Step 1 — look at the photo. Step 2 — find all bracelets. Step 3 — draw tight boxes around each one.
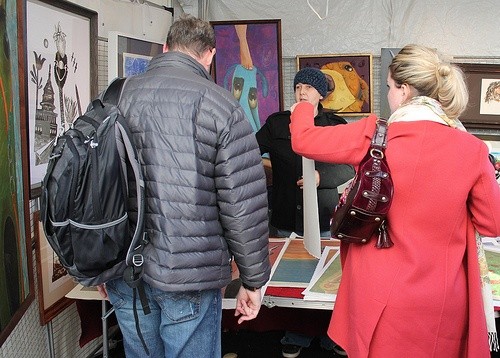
[240,280,262,292]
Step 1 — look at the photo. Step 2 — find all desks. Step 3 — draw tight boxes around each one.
[102,300,335,358]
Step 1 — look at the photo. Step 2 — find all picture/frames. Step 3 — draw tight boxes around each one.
[117,34,164,78]
[24,0,99,200]
[472,134,500,184]
[296,52,374,117]
[209,18,284,160]
[33,210,75,326]
[0,0,36,350]
[449,62,500,130]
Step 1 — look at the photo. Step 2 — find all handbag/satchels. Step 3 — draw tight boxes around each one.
[330,118,395,249]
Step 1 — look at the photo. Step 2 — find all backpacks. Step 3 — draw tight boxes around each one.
[40,76,144,286]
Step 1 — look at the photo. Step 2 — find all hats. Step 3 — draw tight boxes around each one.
[293,68,327,97]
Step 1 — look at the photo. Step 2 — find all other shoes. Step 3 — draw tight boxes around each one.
[282,345,302,358]
[333,345,347,355]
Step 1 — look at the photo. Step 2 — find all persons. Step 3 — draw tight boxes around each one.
[255,66,355,358]
[289,43,500,358]
[85,17,271,358]
